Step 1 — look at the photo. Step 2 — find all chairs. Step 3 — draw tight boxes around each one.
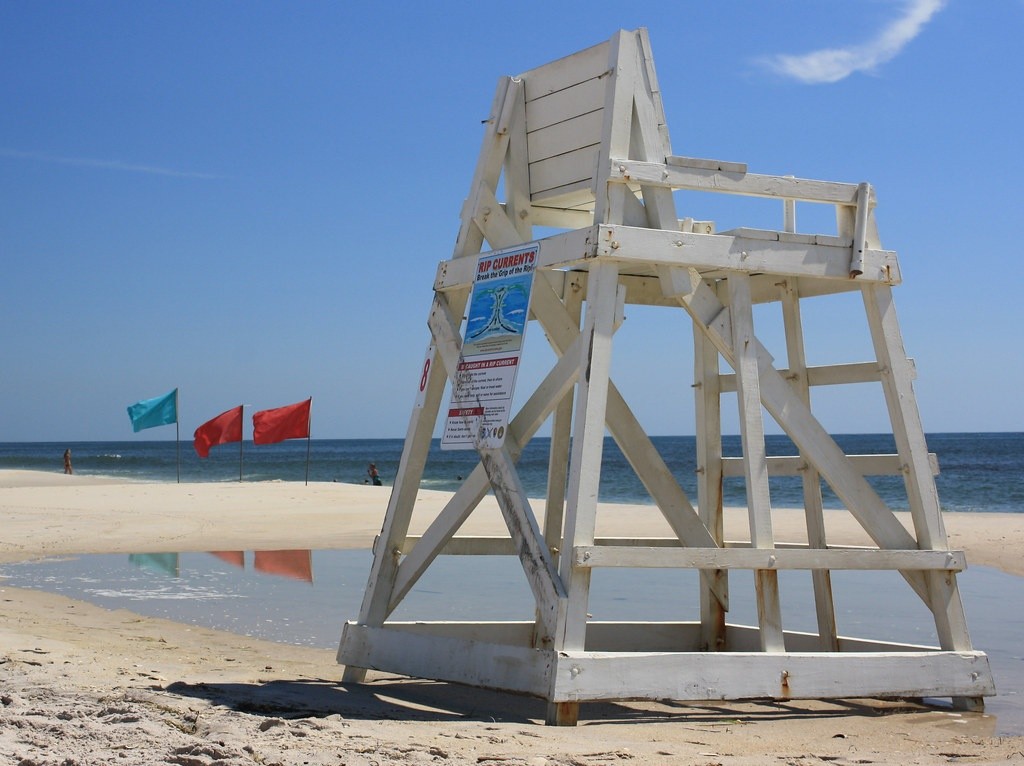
[447,26,880,307]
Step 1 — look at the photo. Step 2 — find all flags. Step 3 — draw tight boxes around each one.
[127,390,177,433]
[130,552,177,577]
[193,405,243,458]
[252,399,310,445]
[254,550,311,580]
[214,550,243,565]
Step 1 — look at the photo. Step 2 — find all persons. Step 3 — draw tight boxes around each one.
[64,448,72,474]
[367,463,382,486]
